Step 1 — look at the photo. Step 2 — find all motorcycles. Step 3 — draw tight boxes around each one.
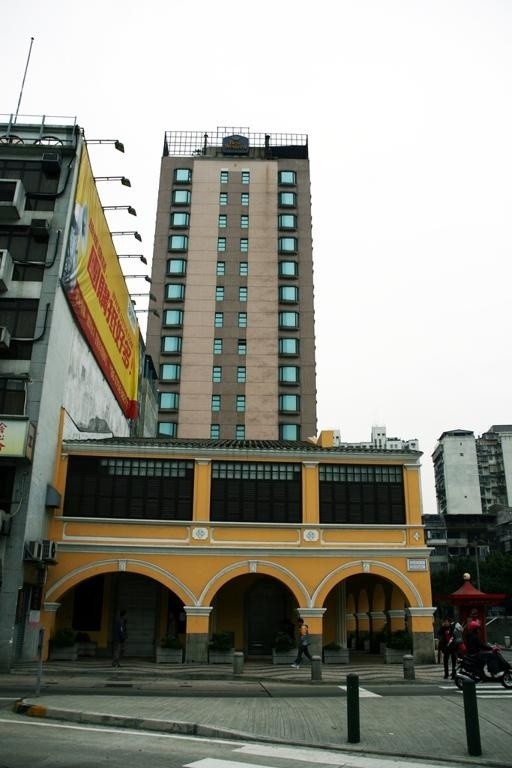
[450,639,512,692]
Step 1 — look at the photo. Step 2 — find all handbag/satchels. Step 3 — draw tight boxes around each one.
[301,627,312,646]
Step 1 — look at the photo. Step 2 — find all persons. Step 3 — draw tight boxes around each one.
[291,617,313,667]
[437,614,459,679]
[463,621,505,678]
[111,607,128,667]
[451,615,465,642]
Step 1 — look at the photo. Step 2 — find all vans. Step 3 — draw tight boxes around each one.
[484,615,512,645]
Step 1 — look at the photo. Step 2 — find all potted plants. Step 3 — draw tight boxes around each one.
[384,634,413,664]
[155,631,236,664]
[272,632,349,665]
[48,628,97,661]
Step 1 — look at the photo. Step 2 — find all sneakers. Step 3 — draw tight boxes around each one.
[289,664,299,669]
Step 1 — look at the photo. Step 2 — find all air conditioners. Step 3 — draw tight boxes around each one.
[29,539,58,562]
